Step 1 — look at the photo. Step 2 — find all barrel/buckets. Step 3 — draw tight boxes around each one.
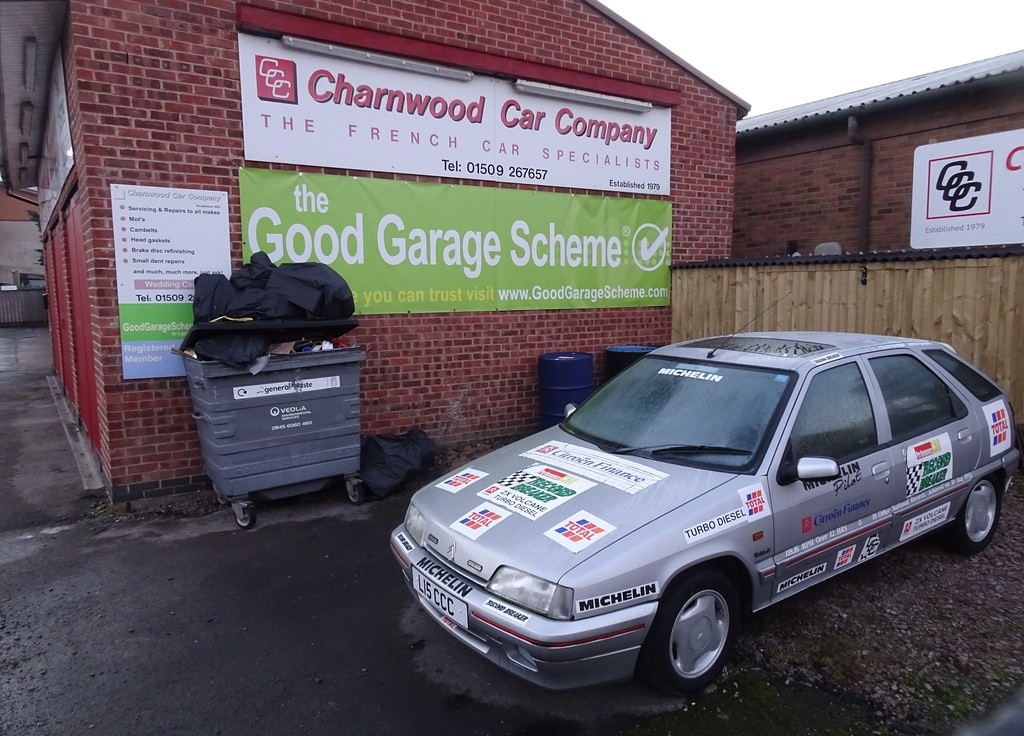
[539,352,595,431]
[604,346,658,383]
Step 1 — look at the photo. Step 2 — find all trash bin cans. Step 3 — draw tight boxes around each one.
[182,342,367,530]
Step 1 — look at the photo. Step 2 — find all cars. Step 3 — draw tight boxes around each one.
[391,326,1020,697]
